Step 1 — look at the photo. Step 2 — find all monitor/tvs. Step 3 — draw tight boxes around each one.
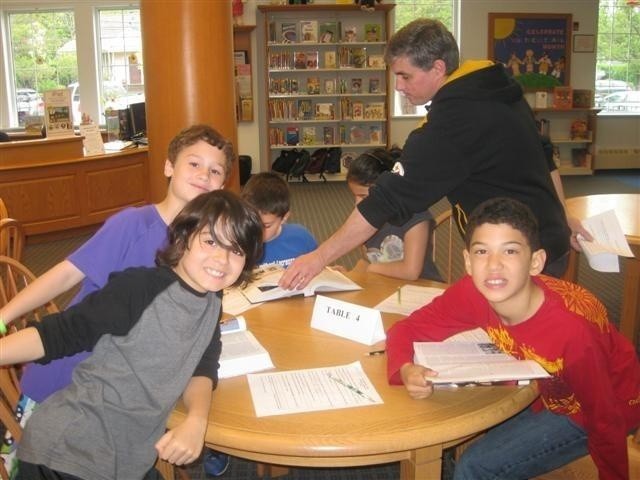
[129,102,146,134]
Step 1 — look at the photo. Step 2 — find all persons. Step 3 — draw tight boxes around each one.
[330,146,444,283]
[0,190,264,480]
[385,196,640,480]
[201,171,320,477]
[276,18,594,290]
[0,124,234,480]
[493,49,565,79]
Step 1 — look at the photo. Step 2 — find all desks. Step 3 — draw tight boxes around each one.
[155,268,539,480]
[561,192,640,341]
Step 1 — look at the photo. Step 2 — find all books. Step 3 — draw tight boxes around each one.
[410,339,554,388]
[217,316,277,380]
[237,264,364,305]
[266,19,386,145]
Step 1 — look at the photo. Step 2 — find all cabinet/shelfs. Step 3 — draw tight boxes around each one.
[528,108,595,175]
[257,4,395,183]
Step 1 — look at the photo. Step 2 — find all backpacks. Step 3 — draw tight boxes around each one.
[272,147,341,184]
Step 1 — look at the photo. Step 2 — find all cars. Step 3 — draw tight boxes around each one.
[15,89,45,115]
[594,80,640,114]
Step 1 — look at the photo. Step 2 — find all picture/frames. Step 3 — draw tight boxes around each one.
[573,34,595,53]
[487,12,573,89]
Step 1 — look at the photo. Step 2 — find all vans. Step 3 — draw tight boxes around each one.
[68,81,126,114]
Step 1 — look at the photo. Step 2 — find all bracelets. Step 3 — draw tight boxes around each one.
[0,319,7,335]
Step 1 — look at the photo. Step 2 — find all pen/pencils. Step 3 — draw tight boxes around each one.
[329,374,375,403]
[398,286,400,304]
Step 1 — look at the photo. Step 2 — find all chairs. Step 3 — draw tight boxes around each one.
[431,208,467,285]
[0,254,138,480]
[0,361,186,480]
[453,432,640,480]
[0,197,28,302]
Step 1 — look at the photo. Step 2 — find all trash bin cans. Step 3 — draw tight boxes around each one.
[238,155,252,185]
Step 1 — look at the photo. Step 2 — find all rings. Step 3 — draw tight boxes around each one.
[298,276,305,282]
